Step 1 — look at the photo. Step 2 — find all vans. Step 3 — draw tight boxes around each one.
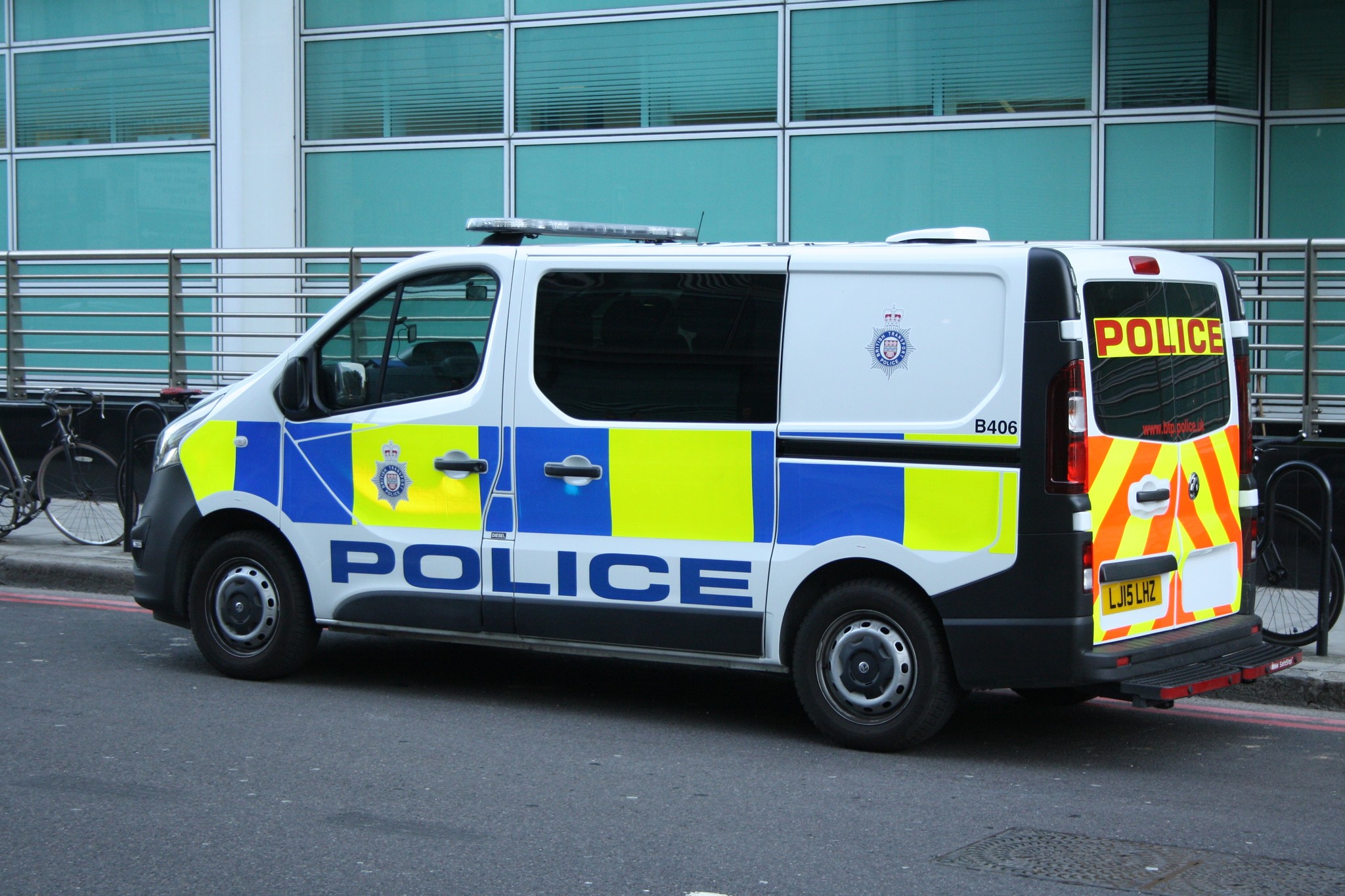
[121,215,1300,754]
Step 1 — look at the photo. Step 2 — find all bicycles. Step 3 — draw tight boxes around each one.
[114,388,202,528]
[0,387,139,546]
[1252,442,1345,649]
